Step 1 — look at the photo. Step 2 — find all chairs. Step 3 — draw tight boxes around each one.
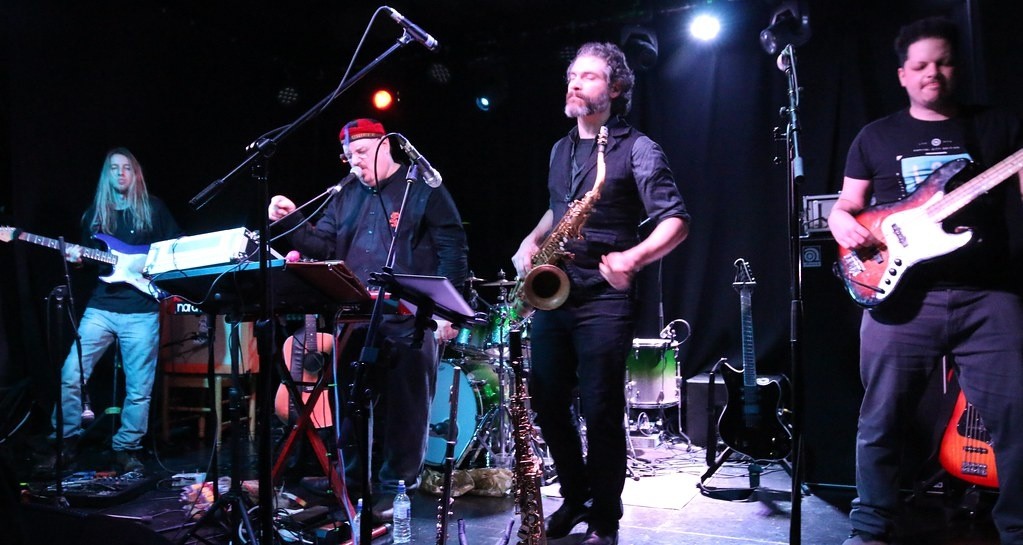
[164,376,256,441]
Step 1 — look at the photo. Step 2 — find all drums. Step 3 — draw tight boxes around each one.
[448,312,489,356]
[484,302,531,359]
[423,355,510,468]
[624,337,678,410]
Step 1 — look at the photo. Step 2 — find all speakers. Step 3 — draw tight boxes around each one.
[161,299,261,376]
[795,238,951,496]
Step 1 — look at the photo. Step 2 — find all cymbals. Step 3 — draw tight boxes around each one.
[465,277,487,281]
[479,280,517,286]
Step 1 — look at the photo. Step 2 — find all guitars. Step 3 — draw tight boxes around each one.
[938,368,1000,488]
[274,314,334,430]
[717,258,793,462]
[837,148,1023,311]
[0,225,171,299]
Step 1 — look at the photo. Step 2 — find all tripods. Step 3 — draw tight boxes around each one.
[175,293,285,545]
[455,284,546,473]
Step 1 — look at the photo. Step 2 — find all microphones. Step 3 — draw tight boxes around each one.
[332,166,363,195]
[776,45,797,71]
[660,322,674,338]
[431,422,448,436]
[395,133,443,188]
[381,6,438,51]
[81,380,94,423]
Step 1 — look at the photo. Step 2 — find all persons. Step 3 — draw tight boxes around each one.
[33,147,185,473]
[268,117,467,522]
[512,42,690,545]
[827,17,1023,545]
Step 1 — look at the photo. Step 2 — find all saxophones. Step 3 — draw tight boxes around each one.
[508,126,609,319]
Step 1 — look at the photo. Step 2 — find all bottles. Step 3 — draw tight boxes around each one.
[393,481,411,543]
[353,506,362,545]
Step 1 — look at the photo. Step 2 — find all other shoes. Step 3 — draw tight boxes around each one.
[579,523,619,545]
[114,450,145,474]
[38,447,76,471]
[841,528,885,545]
[302,474,371,502]
[542,493,601,540]
[368,492,412,522]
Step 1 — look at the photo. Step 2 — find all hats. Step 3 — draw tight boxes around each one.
[340,119,386,143]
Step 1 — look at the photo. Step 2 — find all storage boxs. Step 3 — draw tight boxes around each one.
[139,225,279,277]
